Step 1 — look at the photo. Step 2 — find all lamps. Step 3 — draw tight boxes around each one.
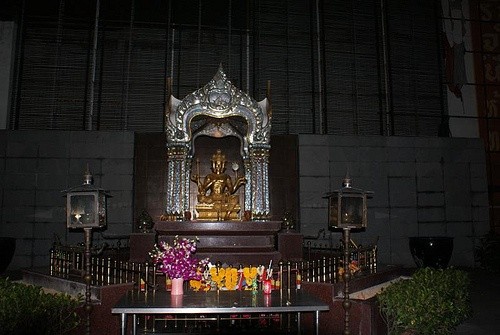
[60,162,112,335]
[321,167,374,335]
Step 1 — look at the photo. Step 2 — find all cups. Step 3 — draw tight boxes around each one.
[262,280,272,295]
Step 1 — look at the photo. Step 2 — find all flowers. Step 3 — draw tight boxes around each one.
[149,235,210,280]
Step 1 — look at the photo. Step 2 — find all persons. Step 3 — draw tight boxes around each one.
[194,150,248,220]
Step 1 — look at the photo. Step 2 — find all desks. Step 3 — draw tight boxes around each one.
[111,289,331,335]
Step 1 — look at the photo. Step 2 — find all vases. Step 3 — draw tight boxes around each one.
[170,277,184,295]
